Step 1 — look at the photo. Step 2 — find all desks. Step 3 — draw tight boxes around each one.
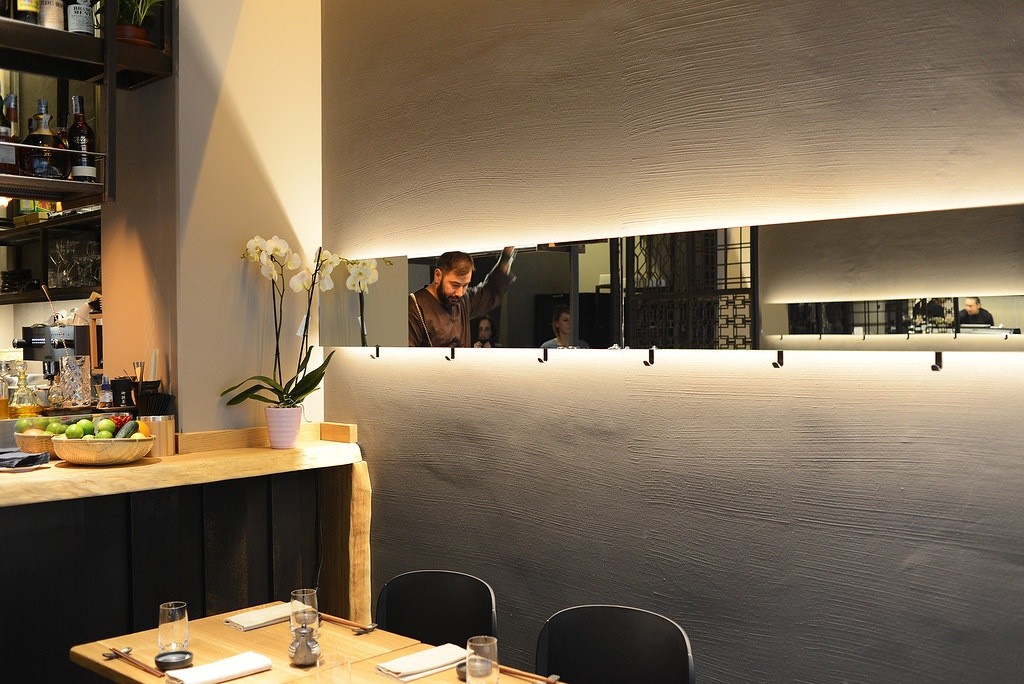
[285,642,545,684]
[69,601,421,684]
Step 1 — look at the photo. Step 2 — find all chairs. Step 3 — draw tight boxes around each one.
[535,604,695,684]
[376,570,498,651]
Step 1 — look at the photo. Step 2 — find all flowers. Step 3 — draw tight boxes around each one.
[346,258,394,347]
[220,235,349,406]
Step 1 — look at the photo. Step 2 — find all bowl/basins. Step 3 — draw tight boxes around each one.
[456,663,466,680]
[295,610,316,623]
[155,650,193,669]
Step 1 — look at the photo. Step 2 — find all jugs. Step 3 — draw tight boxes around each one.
[288,623,321,665]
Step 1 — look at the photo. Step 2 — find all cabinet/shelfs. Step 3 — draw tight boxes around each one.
[0,0,172,305]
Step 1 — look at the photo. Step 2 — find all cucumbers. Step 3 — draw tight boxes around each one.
[115,421,139,437]
[60,415,93,424]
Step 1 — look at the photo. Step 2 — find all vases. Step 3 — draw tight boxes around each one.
[264,406,303,449]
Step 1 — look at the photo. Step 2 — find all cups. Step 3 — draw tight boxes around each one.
[136,416,176,457]
[466,636,500,684]
[290,589,319,640]
[132,380,161,406]
[60,355,91,408]
[158,601,188,653]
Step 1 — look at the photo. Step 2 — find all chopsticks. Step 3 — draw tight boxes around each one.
[138,393,176,416]
[123,362,145,382]
[320,614,368,632]
[109,647,164,678]
[500,664,547,682]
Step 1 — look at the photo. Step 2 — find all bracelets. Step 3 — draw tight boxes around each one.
[500,253,514,263]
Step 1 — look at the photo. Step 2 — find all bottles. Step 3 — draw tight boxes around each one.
[0,0,94,36]
[46,238,101,290]
[97,376,113,409]
[0,361,43,420]
[0,93,96,182]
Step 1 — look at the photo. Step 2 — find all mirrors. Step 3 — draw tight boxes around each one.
[319,204,1024,351]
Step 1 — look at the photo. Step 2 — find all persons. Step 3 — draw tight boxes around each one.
[958,297,994,326]
[474,315,502,348]
[913,298,946,318]
[407,247,515,349]
[541,305,589,349]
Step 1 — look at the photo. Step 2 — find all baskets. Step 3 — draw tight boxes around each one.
[14,431,61,459]
[51,434,156,465]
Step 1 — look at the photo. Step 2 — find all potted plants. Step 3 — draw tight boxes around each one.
[94,1,164,49]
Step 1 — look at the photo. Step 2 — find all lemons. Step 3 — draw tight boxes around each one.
[15,417,67,435]
[57,419,150,440]
